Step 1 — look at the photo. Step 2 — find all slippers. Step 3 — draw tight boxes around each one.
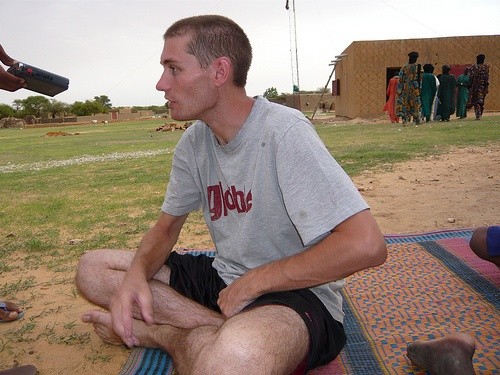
[0,301,24,323]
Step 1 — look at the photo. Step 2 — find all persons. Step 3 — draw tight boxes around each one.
[406,333,476,375]
[75,15,388,375]
[0,36,26,324]
[383,51,489,126]
[468,226,500,266]
